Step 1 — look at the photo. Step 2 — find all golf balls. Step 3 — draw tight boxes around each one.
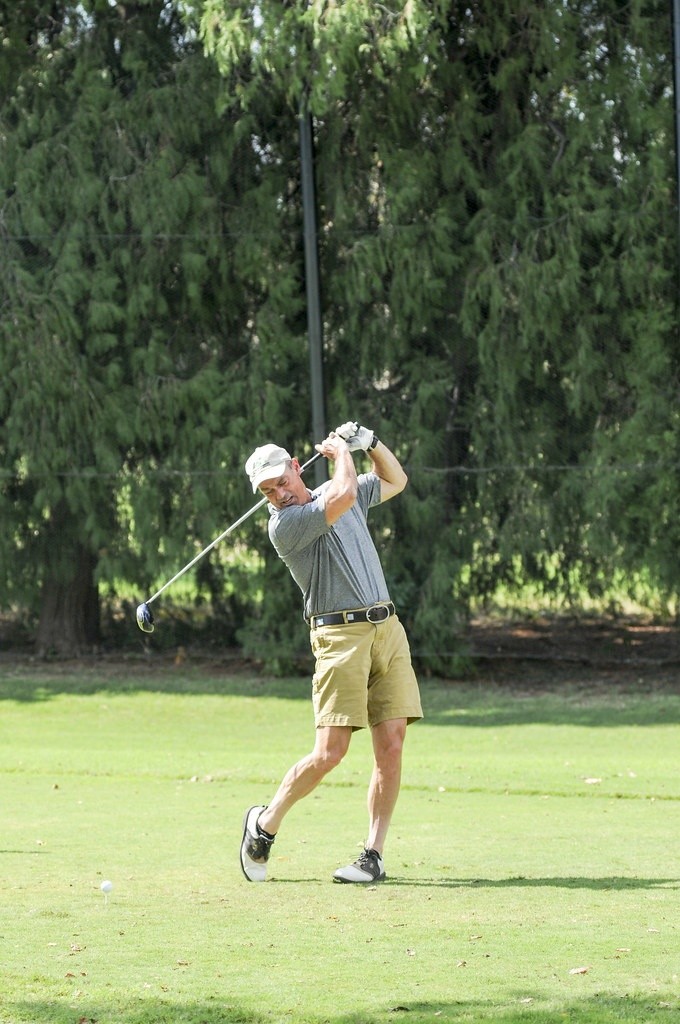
[101,880,113,893]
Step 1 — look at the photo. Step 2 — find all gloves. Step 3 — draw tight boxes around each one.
[329,421,374,452]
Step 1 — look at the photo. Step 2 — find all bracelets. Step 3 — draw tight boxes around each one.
[366,435,378,451]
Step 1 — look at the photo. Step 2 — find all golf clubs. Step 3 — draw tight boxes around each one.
[137,421,361,633]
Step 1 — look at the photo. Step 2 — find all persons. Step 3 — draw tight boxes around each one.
[240,421,424,883]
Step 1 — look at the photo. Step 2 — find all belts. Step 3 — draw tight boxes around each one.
[315,604,394,627]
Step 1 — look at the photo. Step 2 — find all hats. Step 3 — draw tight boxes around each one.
[245,444,292,495]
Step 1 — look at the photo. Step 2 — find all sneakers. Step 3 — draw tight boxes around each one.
[333,851,387,884]
[239,804,275,882]
